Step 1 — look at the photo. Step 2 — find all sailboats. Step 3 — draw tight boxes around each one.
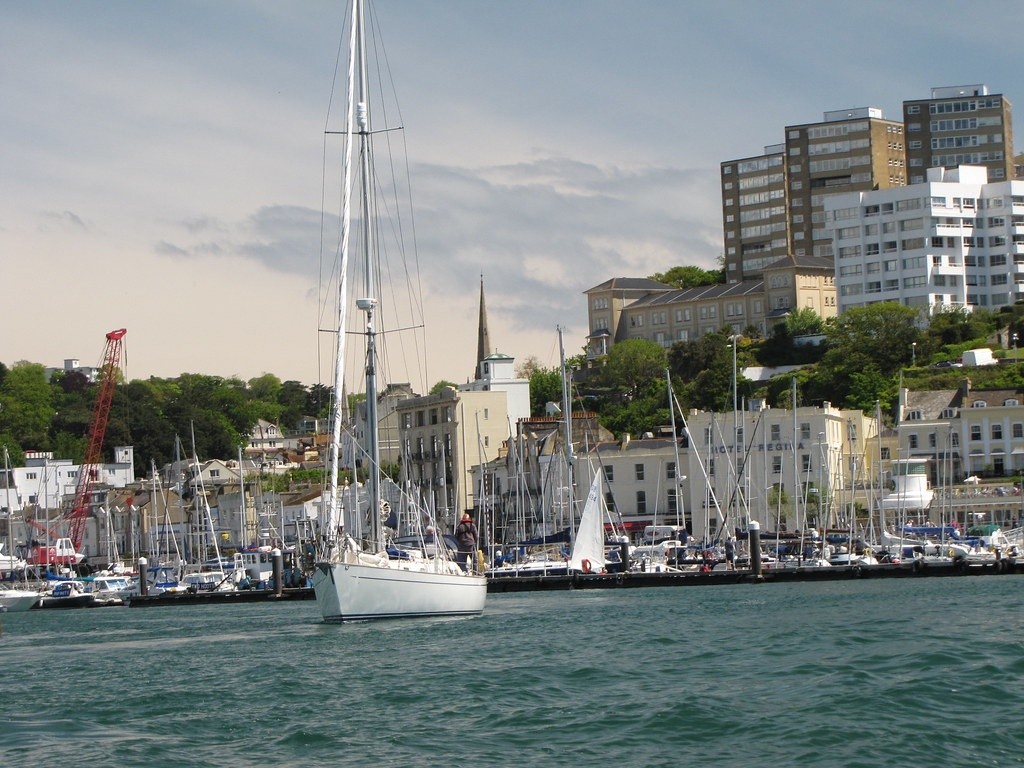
[0,325,1024,606]
[309,0,486,619]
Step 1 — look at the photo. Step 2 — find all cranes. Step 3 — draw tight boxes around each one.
[22,328,136,564]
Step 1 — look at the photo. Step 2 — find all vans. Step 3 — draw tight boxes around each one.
[642,525,685,545]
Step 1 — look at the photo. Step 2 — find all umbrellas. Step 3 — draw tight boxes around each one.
[963,476,982,482]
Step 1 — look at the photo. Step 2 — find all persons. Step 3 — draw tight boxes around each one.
[725,536,737,570]
[771,547,791,560]
[1000,486,1006,495]
[455,514,483,576]
